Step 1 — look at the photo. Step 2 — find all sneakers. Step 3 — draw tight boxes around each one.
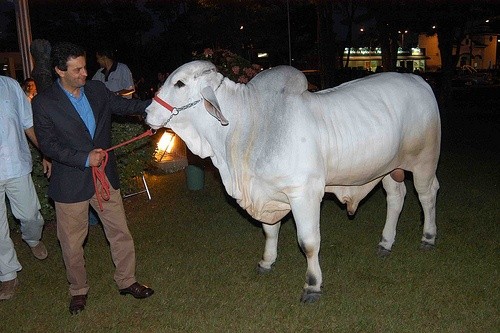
[0,278,15,300]
[31,241,47,259]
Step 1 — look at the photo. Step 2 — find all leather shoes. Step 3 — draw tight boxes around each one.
[119,282,154,299]
[69,294,88,315]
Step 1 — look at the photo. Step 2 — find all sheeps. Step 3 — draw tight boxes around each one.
[144,60,441,303]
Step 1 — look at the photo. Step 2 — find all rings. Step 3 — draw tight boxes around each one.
[98,163,101,166]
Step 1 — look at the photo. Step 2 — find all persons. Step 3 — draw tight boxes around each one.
[22,78,37,102]
[31,42,166,315]
[0,76,53,300]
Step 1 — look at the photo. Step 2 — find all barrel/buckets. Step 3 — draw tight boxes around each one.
[185,164,206,191]
